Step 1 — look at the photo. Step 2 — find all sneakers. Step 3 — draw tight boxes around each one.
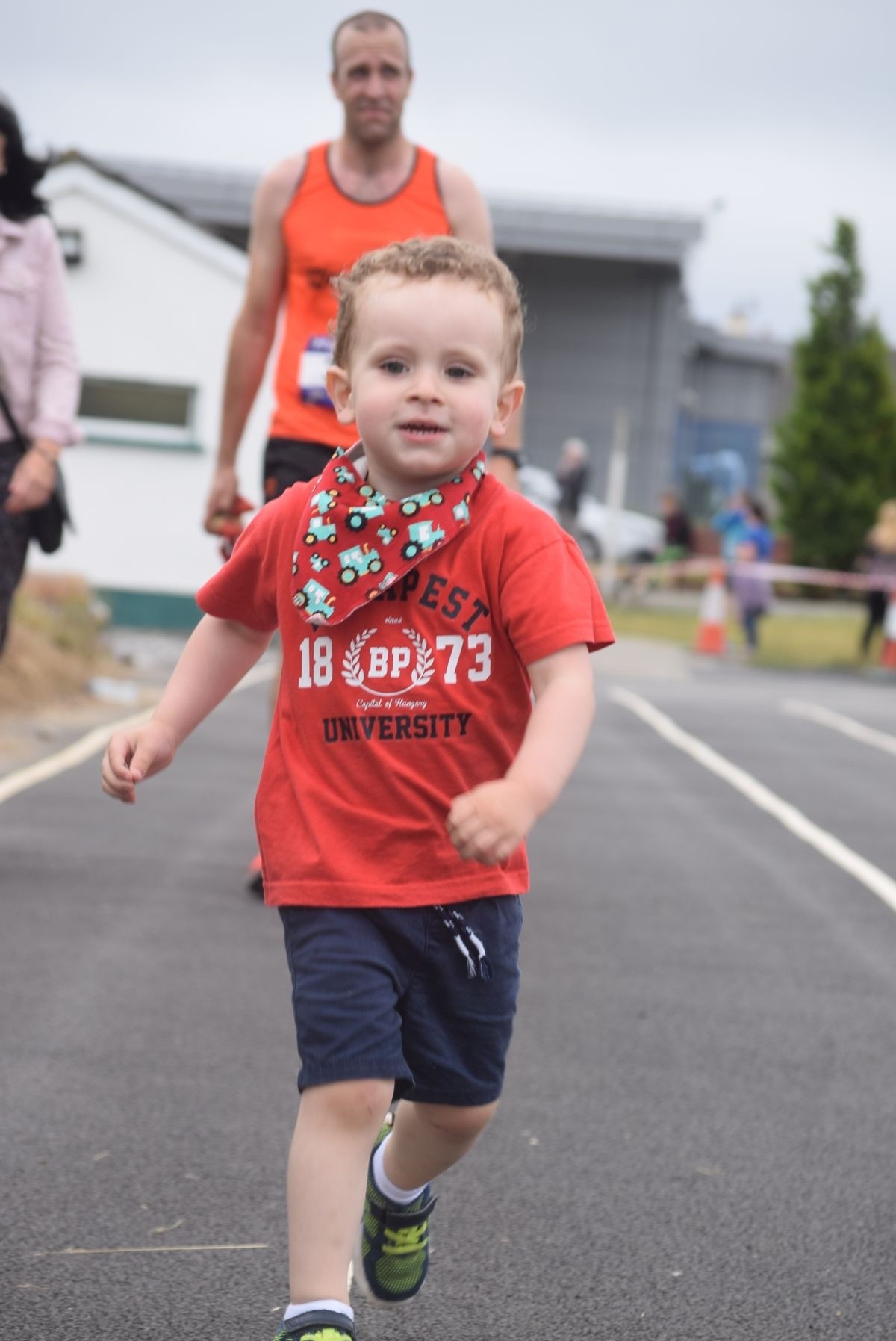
[272,1301,356,1341]
[353,1113,440,1304]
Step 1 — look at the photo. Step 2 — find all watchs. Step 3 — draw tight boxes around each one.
[488,445,527,469]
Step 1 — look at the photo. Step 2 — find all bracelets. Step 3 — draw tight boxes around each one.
[31,444,58,468]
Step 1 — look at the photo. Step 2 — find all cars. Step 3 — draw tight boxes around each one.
[518,462,669,567]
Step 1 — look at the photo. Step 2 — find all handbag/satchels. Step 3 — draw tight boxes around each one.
[1,391,71,554]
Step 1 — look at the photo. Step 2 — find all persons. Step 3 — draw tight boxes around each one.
[854,497,895,671]
[616,547,660,597]
[723,536,779,655]
[0,94,84,664]
[101,231,617,1341]
[649,490,698,597]
[196,7,528,904]
[709,486,752,596]
[552,435,591,540]
[727,499,779,566]
